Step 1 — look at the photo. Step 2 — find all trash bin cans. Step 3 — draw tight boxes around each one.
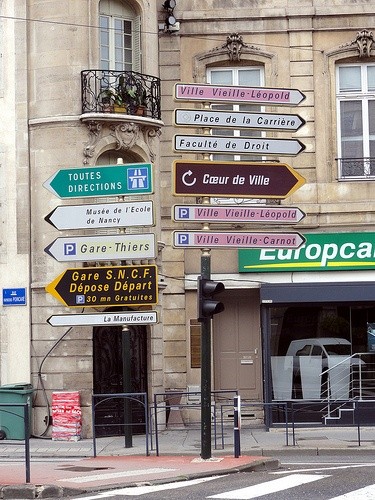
[0,383,34,440]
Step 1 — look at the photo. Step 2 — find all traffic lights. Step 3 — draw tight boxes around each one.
[195,279,226,322]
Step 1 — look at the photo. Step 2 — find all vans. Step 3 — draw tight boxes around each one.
[285,338,369,380]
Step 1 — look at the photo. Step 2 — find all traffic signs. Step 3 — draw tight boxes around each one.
[43,264,159,309]
[173,108,308,133]
[41,163,155,201]
[170,158,308,199]
[171,230,311,253]
[170,203,306,226]
[45,200,157,231]
[173,82,306,106]
[172,134,307,158]
[46,310,158,327]
[44,232,159,263]
